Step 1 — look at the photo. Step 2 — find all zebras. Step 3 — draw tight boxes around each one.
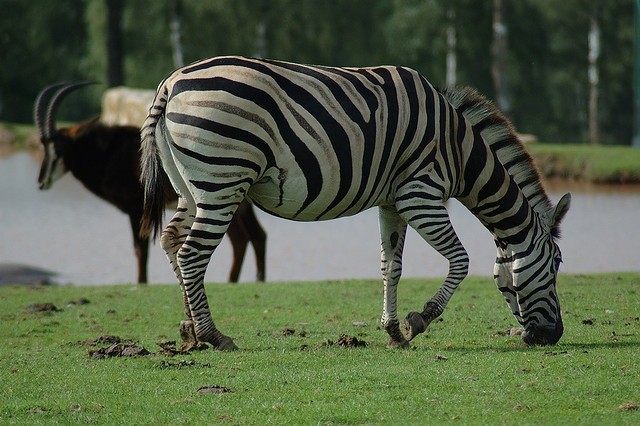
[137,53,572,352]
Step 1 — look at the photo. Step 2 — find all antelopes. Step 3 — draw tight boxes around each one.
[32,79,267,281]
